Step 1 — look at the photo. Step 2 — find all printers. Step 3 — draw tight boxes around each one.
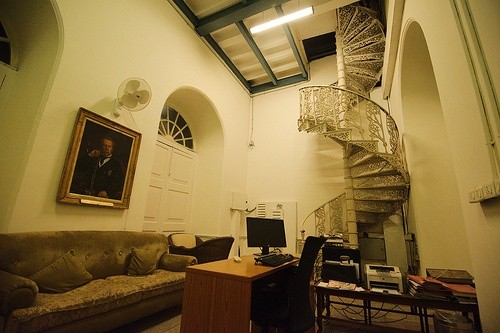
[363,263,403,293]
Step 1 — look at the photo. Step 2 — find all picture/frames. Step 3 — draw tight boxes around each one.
[56,108,142,209]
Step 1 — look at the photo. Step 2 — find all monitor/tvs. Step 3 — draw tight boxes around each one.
[245,216,287,258]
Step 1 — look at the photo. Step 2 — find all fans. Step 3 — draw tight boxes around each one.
[114,77,152,119]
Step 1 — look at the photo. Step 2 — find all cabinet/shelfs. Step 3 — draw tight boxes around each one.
[322,247,362,283]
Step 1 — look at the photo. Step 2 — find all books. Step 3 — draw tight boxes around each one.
[407,274,477,301]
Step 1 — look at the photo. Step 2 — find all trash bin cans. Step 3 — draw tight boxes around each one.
[433,311,473,333]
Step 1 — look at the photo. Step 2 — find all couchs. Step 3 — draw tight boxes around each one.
[0,231,198,333]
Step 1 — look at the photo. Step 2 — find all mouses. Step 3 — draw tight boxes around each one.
[285,253,293,258]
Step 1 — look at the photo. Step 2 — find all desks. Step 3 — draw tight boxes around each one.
[313,279,482,333]
[179,255,300,333]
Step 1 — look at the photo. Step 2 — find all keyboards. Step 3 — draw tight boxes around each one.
[255,255,294,265]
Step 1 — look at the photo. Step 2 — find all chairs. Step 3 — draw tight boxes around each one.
[167,233,235,264]
[251,235,327,333]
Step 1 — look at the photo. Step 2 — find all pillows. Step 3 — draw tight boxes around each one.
[171,234,196,248]
[28,250,93,294]
[128,248,159,275]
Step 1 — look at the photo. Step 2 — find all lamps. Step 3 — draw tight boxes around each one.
[249,6,314,34]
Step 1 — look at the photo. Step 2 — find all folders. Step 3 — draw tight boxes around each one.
[406,275,453,301]
